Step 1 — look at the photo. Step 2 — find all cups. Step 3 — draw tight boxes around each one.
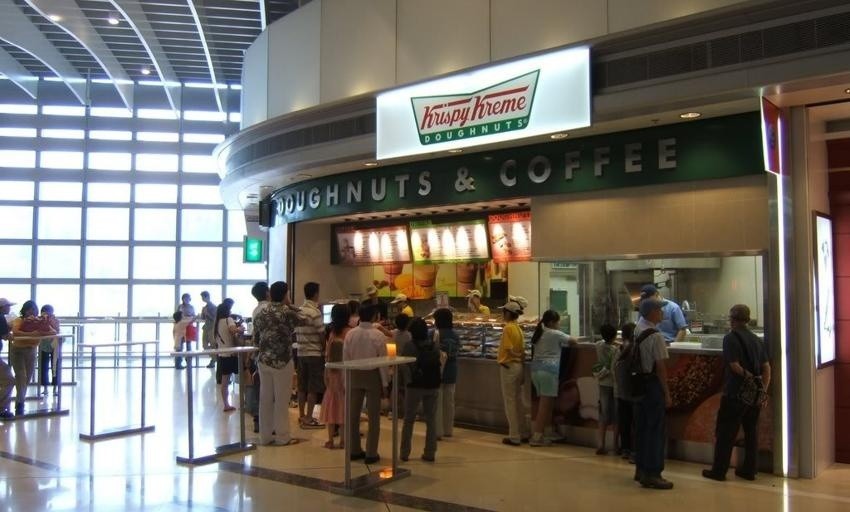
[383,264,403,290]
[410,264,440,297]
[385,342,397,360]
[454,264,478,292]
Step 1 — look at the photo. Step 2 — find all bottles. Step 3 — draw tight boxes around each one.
[247,317,253,331]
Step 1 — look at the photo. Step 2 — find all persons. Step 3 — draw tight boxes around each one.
[0,296,19,422]
[193,290,218,368]
[593,322,622,457]
[7,299,57,415]
[527,310,581,448]
[173,311,201,370]
[635,283,688,342]
[631,297,675,489]
[700,302,773,482]
[176,293,197,369]
[214,281,525,465]
[37,303,61,398]
[609,322,640,463]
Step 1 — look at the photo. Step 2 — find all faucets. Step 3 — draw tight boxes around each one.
[681,299,690,311]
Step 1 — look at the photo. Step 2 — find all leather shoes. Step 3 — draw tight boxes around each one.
[701,469,726,482]
[735,467,756,482]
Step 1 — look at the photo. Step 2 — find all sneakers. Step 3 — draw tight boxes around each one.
[0,411,13,419]
[594,445,639,464]
[640,476,673,489]
[15,408,24,416]
[502,432,566,447]
[297,415,380,465]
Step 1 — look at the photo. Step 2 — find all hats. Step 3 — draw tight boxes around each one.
[496,302,519,313]
[468,288,482,298]
[365,284,378,296]
[0,298,16,306]
[637,284,658,300]
[390,294,408,304]
[640,299,670,314]
[509,295,528,308]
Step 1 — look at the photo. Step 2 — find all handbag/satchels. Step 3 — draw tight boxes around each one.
[590,361,612,380]
[186,325,198,342]
[233,328,243,345]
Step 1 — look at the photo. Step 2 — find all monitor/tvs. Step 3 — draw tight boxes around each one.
[323,303,335,324]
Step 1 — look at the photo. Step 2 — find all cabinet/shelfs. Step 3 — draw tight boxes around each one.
[422,308,572,361]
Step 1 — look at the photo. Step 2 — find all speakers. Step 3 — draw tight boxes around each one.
[259,201,270,226]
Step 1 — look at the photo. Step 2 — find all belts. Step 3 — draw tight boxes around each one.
[500,362,510,370]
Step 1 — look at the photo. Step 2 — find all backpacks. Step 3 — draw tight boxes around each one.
[439,350,447,375]
[612,329,660,403]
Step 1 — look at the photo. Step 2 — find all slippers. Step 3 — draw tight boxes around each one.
[223,406,235,412]
[266,439,275,444]
[279,438,300,446]
